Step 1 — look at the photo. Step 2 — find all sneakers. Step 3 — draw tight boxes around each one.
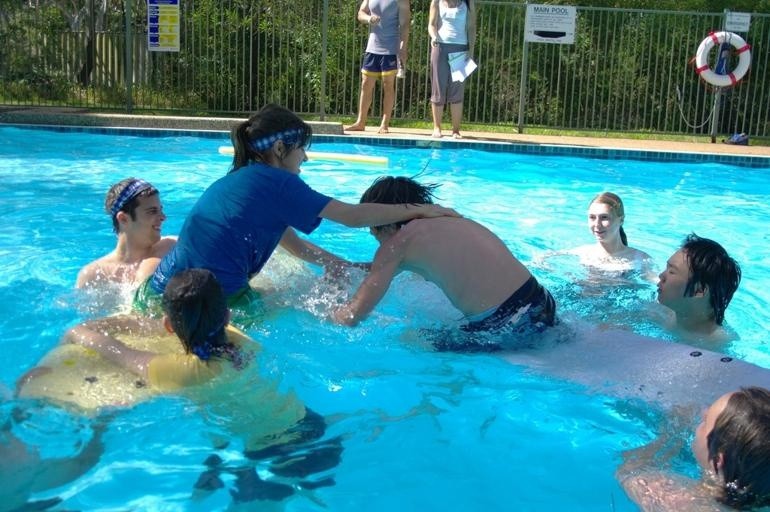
[721,132,750,146]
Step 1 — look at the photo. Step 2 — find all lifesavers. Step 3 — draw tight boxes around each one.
[692,31,752,87]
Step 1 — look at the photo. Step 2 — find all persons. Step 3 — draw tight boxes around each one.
[131,105,460,371]
[63,270,345,511]
[325,176,576,354]
[427,0,476,139]
[568,191,660,286]
[616,386,770,512]
[645,231,742,349]
[77,177,179,286]
[343,0,410,134]
